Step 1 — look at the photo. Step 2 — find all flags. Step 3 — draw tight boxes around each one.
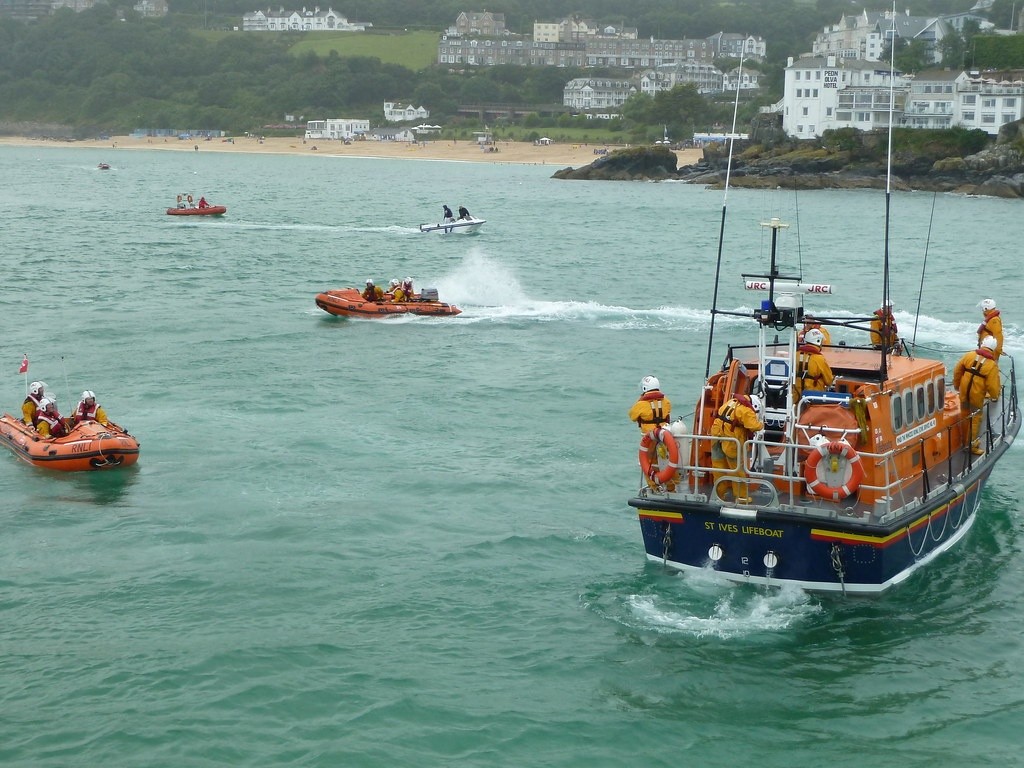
[19,358,28,373]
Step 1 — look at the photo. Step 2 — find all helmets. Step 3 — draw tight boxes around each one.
[40,398,56,411]
[405,277,413,285]
[366,279,373,286]
[82,390,95,404]
[641,375,659,395]
[804,329,824,346]
[749,395,762,413]
[881,299,895,309]
[981,335,997,351]
[30,381,43,395]
[391,279,399,286]
[981,298,996,312]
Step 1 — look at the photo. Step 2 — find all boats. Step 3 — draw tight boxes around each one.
[97,163,111,169]
[625,0,1024,599]
[0,352,141,472]
[315,287,464,320]
[166,205,226,216]
[419,214,487,235]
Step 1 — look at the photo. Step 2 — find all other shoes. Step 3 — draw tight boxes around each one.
[991,397,997,402]
[985,391,990,399]
[721,494,727,501]
[738,496,753,504]
[669,490,677,494]
[972,448,984,455]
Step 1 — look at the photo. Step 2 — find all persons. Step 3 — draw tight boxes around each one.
[628,375,670,489]
[711,395,765,503]
[177,196,186,209]
[37,398,70,438]
[798,314,830,342]
[363,279,379,302]
[870,299,904,352]
[442,205,452,223]
[70,389,107,429]
[457,205,469,220]
[953,334,1001,455]
[976,299,1004,397]
[389,278,404,303]
[22,380,51,430]
[199,196,211,209]
[186,195,196,209]
[793,329,834,402]
[386,276,416,302]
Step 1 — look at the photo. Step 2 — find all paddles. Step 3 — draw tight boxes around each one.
[0,415,39,441]
[376,302,449,307]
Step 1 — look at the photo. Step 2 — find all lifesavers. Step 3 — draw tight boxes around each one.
[805,442,863,500]
[188,196,192,203]
[178,196,182,202]
[638,428,679,485]
